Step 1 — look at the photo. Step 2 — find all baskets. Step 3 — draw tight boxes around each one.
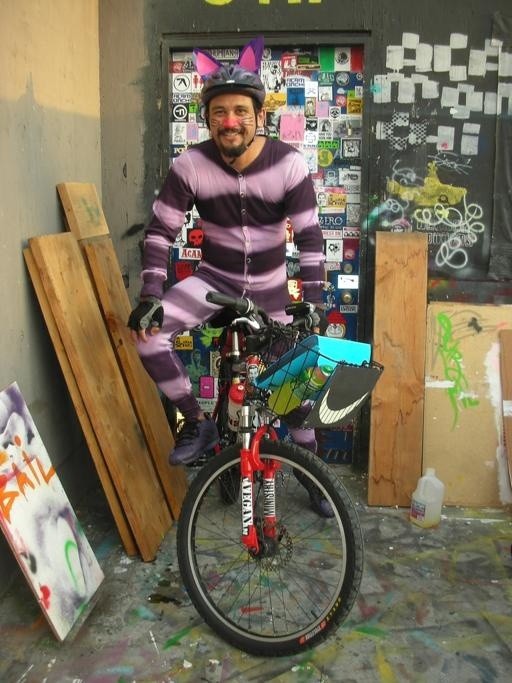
[245,323,383,432]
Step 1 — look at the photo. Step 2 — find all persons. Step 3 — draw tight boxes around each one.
[128,66,334,518]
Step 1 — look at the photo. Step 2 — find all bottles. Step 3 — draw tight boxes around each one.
[268,365,334,415]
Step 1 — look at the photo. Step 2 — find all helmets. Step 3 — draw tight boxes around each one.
[200,65,266,106]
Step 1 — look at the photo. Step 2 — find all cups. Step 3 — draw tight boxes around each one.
[226,384,245,432]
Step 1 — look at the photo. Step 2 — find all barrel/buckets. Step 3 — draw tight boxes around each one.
[409,466,446,528]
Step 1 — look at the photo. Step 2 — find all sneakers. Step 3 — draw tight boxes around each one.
[169,412,220,464]
[293,467,336,516]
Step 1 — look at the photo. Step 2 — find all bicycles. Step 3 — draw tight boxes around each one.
[177,291,384,659]
